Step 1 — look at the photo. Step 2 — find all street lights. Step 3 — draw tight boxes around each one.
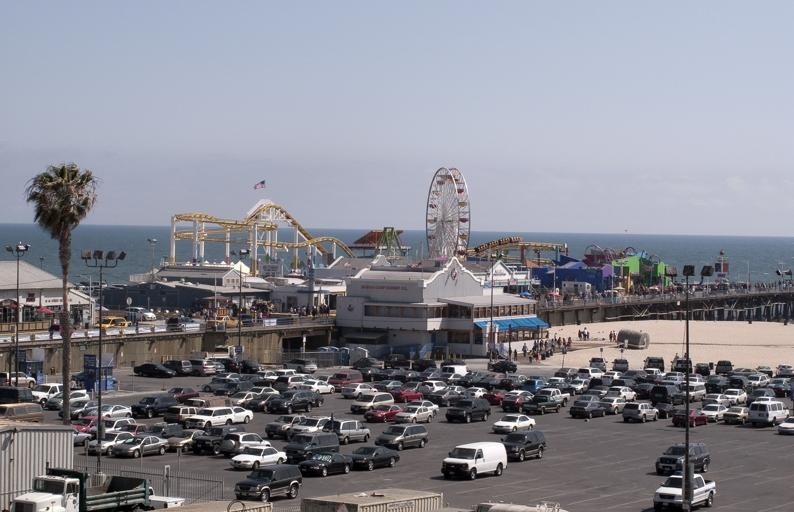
[661,264,719,512]
[229,247,252,347]
[491,252,499,342]
[550,259,556,302]
[79,247,127,472]
[4,239,35,387]
[38,255,46,269]
[719,248,726,284]
[147,237,158,282]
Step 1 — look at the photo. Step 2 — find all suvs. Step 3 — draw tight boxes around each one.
[231,462,305,504]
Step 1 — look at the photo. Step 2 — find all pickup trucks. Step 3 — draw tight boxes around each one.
[145,484,187,512]
[650,471,718,512]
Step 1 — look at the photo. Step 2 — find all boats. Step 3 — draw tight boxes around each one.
[74,277,109,295]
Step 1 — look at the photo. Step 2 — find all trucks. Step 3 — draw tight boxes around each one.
[4,460,153,512]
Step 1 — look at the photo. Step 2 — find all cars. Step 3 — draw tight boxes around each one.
[96,307,249,332]
[518,279,662,303]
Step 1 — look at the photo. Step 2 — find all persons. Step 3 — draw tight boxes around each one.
[579,279,794,303]
[508,328,617,363]
[199,297,328,321]
